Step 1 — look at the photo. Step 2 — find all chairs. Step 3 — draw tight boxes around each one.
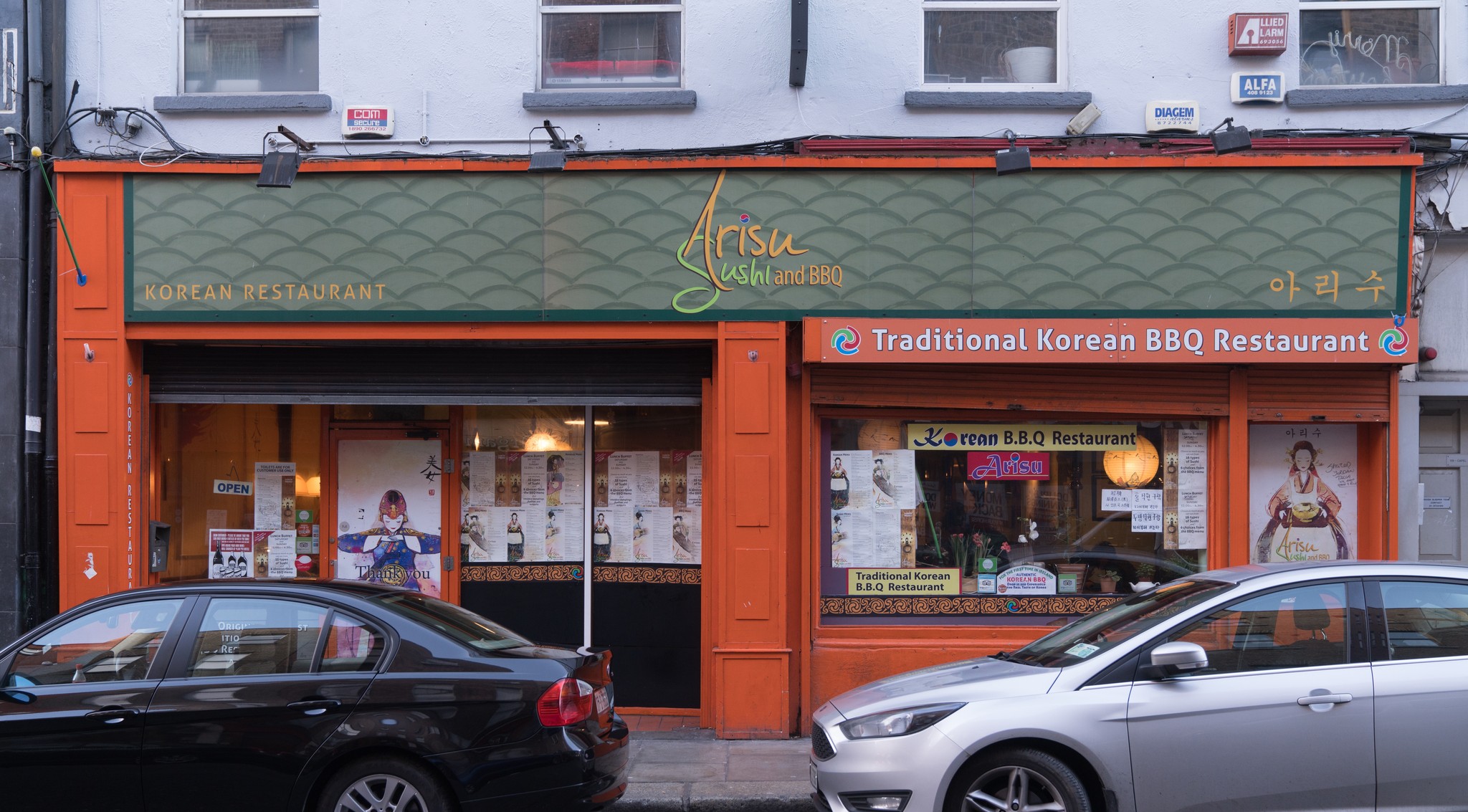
[1270,592,1339,666]
[192,617,235,678]
[236,629,278,676]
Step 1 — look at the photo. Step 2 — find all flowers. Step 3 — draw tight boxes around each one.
[950,531,970,574]
[1014,514,1040,544]
[972,532,1011,570]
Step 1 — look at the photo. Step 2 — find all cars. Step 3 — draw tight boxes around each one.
[961,508,1204,592]
[0,579,632,812]
[808,560,1468,812]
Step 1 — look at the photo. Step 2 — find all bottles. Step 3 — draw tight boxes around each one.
[72,664,86,683]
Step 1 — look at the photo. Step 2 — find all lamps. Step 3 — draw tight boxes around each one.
[523,124,571,175]
[253,141,304,192]
[993,139,1035,179]
[295,470,321,498]
[1206,120,1256,158]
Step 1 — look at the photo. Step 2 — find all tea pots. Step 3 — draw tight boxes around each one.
[1129,582,1160,592]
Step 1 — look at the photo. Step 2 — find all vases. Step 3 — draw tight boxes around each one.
[960,577,978,593]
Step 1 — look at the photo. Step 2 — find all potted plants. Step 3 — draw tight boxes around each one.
[1094,569,1123,595]
[1134,561,1158,583]
[1053,506,1088,591]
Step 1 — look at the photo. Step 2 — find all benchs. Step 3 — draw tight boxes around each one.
[1395,631,1468,661]
